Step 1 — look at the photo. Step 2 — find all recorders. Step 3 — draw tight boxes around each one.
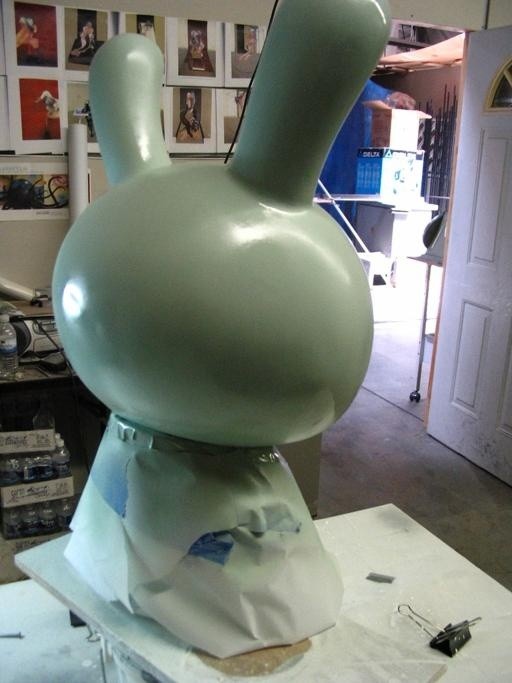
[0,296,66,355]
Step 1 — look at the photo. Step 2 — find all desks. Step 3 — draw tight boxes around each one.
[0,364,111,474]
[13,502,512,683]
[313,193,438,287]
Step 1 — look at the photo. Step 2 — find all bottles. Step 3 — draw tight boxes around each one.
[0,433,73,539]
[0,313,18,378]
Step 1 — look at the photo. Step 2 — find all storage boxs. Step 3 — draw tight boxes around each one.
[362,100,432,149]
[0,429,74,584]
[355,148,425,196]
